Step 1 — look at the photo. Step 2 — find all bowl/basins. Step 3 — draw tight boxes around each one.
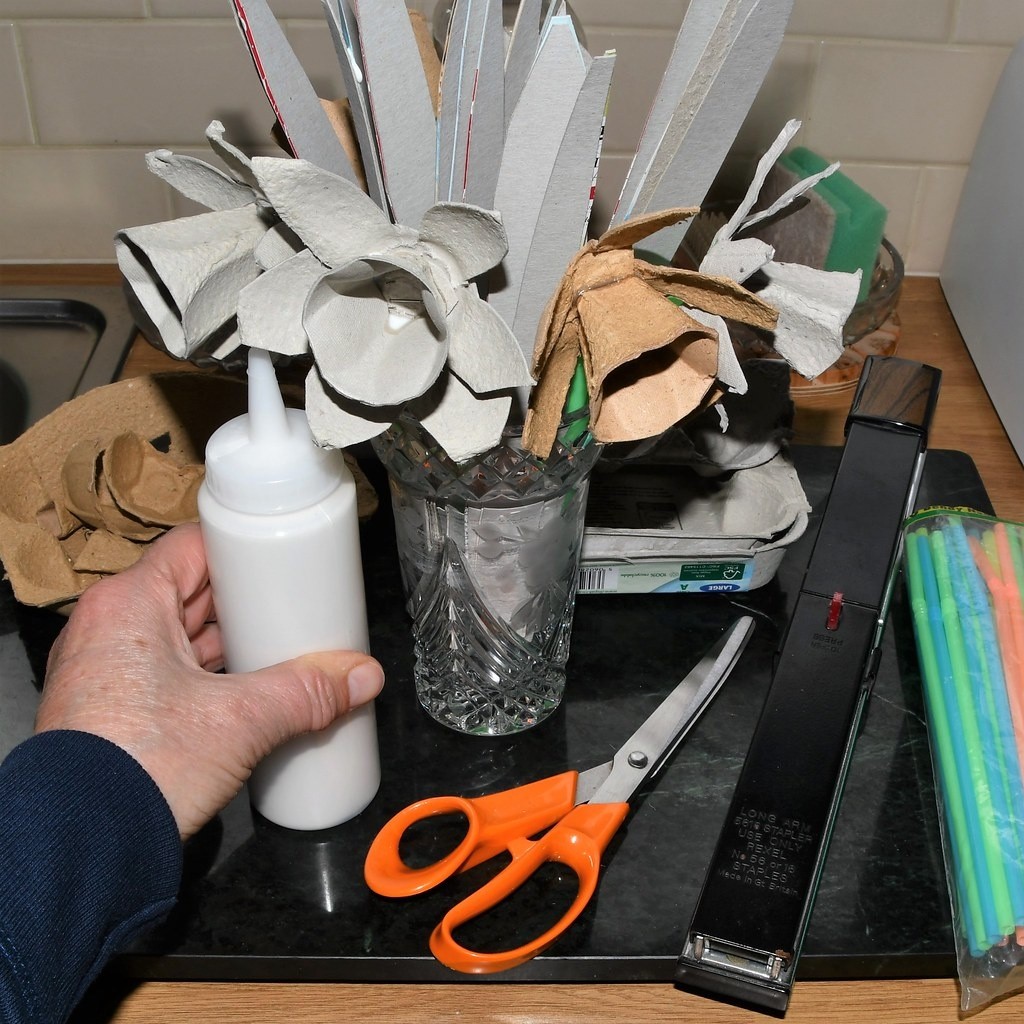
[679,198,904,345]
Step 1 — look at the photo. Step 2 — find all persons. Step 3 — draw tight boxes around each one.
[0,520,384,1024]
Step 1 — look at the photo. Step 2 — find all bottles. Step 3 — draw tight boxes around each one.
[197,348,382,830]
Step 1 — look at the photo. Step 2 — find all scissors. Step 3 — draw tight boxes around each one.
[363,617,761,976]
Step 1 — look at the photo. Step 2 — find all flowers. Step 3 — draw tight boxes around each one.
[114,0,862,463]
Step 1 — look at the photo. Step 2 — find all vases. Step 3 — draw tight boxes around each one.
[368,409,609,737]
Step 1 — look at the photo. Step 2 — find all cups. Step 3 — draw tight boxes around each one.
[371,406,604,736]
